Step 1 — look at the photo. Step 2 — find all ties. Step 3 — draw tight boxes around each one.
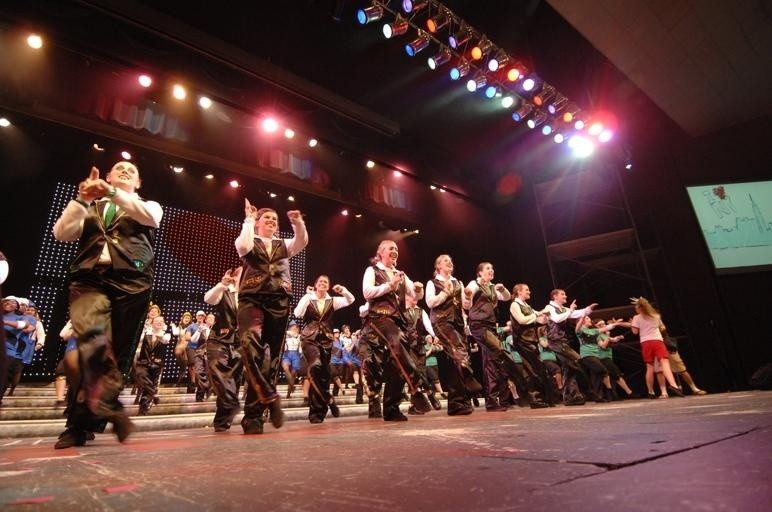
[104,203,116,227]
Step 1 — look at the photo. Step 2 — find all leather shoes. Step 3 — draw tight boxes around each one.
[487,387,707,411]
[112,416,135,443]
[54,428,87,449]
[214,393,479,433]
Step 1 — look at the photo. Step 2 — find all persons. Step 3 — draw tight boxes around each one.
[630,296,707,400]
[1,161,632,451]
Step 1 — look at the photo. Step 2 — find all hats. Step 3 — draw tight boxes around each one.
[196,311,205,316]
[2,296,35,307]
[149,305,161,314]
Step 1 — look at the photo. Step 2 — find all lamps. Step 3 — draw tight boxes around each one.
[402,0,601,132]
[355,0,586,153]
